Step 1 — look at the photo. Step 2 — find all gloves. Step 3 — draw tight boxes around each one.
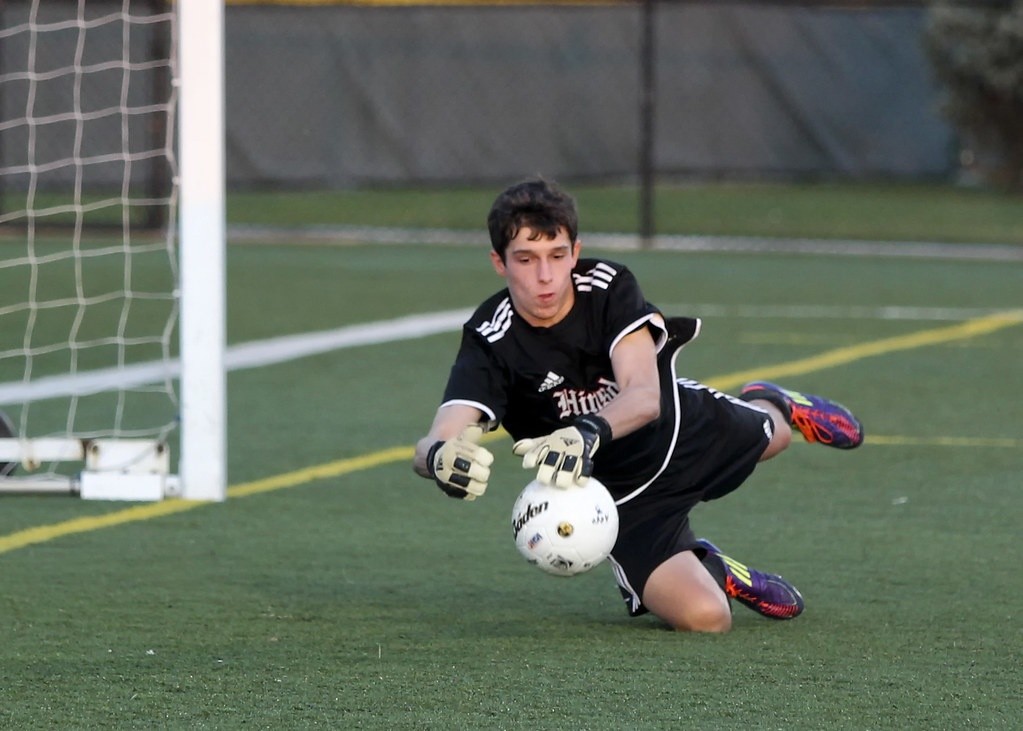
[427,427,494,503]
[512,414,603,488]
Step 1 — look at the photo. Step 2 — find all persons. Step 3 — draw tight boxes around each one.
[412,181,865,634]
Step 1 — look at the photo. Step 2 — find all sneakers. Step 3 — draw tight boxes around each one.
[741,382,863,449]
[696,540,804,620]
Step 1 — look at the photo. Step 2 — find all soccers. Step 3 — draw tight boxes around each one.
[510,475,621,577]
